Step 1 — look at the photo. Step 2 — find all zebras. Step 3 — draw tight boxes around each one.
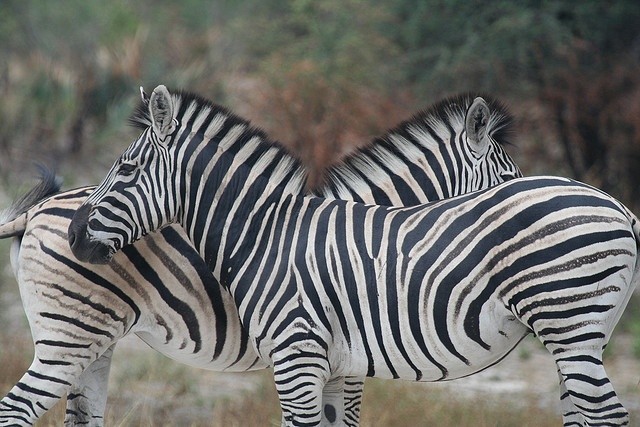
[68,83,640,427]
[0,92,525,427]
